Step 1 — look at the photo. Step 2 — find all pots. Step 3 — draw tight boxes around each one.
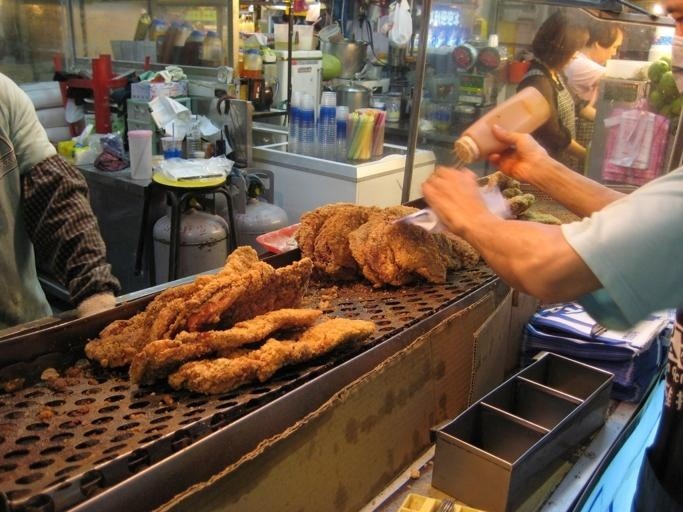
[317,33,370,80]
[323,80,377,113]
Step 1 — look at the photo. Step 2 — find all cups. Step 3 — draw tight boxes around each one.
[345,108,386,161]
[287,89,349,155]
[425,77,452,131]
[161,137,183,159]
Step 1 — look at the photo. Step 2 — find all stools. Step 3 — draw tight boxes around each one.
[134,169,238,282]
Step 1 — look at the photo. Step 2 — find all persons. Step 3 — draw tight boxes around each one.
[0,69,119,333]
[420,124,683,332]
[513,9,623,173]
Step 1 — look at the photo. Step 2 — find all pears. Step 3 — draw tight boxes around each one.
[321,54,342,82]
[646,57,683,118]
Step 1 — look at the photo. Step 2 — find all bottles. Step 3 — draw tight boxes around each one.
[127,129,153,180]
[368,95,385,111]
[148,17,222,68]
[241,48,263,100]
[384,92,402,122]
[453,85,551,165]
[186,118,201,159]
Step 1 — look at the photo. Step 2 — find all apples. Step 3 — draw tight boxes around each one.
[477,50,498,70]
[452,47,471,68]
